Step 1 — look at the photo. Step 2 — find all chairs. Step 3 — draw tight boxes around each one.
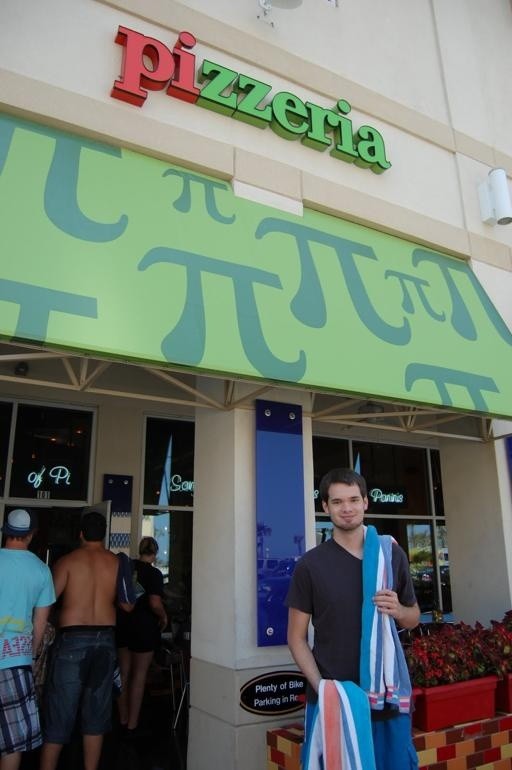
[166,619,191,733]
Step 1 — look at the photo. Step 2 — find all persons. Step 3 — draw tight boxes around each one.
[285,466,422,770]
[118,535,167,735]
[45,511,137,769]
[1,504,54,769]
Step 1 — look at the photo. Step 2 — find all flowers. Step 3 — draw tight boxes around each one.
[401,611,511,686]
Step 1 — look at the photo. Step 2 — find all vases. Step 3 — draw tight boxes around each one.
[414,677,502,731]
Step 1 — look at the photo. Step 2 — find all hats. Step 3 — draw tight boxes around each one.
[7,509,32,531]
